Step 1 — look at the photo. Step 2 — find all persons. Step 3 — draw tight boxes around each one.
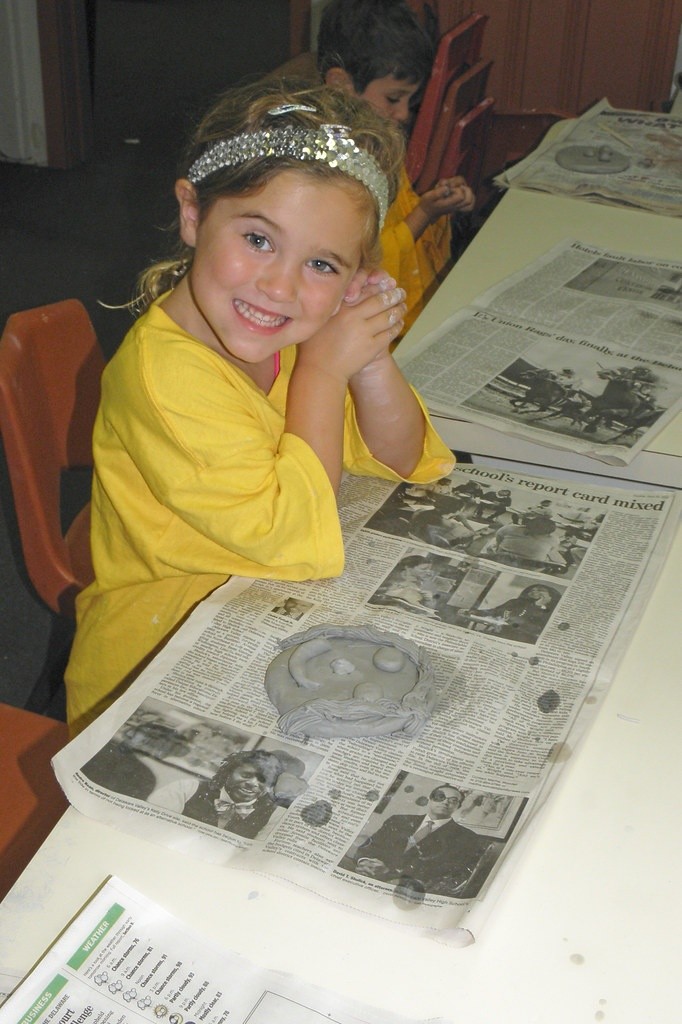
[271,595,313,621]
[550,363,671,423]
[310,0,474,365]
[369,467,602,648]
[63,76,458,755]
[147,748,288,842]
[353,782,486,899]
[80,738,157,802]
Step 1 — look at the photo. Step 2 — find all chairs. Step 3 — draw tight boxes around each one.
[402,12,494,245]
[0,299,105,711]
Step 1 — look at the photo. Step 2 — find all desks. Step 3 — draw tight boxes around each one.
[0,97,681,1024]
